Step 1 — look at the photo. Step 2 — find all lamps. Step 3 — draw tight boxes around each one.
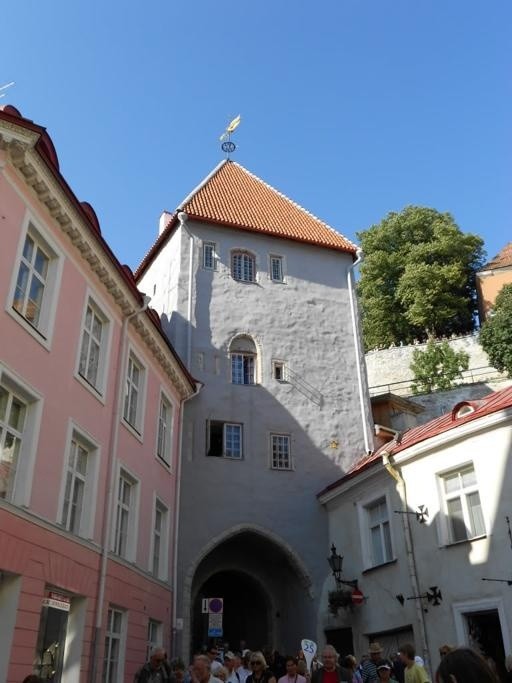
[326,542,358,592]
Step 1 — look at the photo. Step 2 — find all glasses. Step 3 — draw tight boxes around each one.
[440,652,446,656]
[251,661,262,665]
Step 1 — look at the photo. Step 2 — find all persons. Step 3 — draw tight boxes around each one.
[133,636,512,683]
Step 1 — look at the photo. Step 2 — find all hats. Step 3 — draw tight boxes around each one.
[376,664,392,670]
[368,642,384,653]
[224,651,235,661]
